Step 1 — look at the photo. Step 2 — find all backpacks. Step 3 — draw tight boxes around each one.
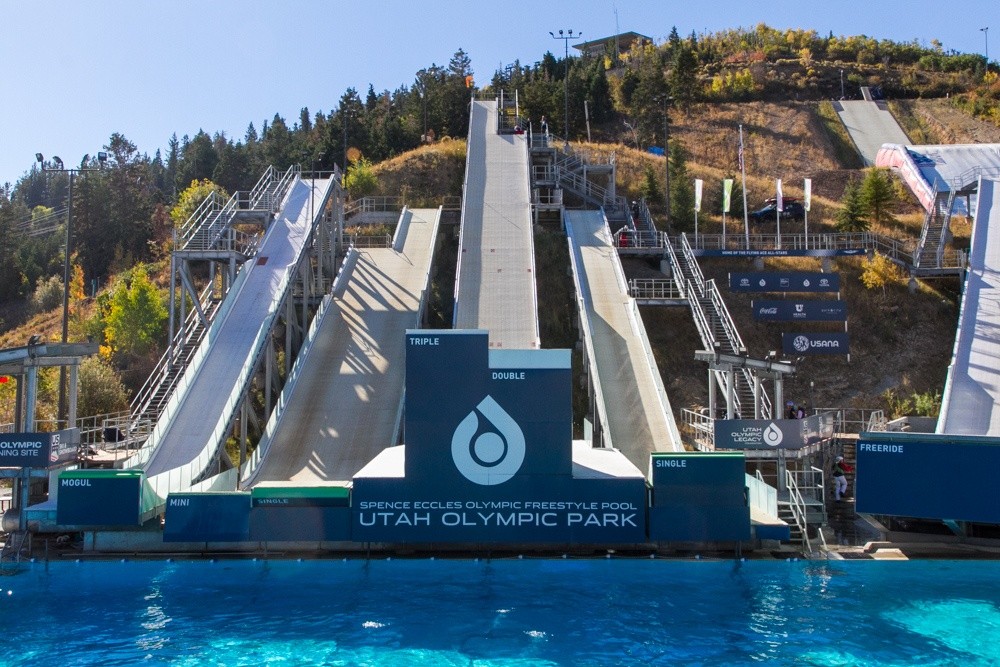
[831,462,844,477]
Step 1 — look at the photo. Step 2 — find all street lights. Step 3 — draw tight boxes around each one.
[652,94,673,235]
[548,28,582,146]
[35,151,109,430]
[979,27,989,95]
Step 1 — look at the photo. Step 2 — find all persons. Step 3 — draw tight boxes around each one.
[425,126,451,144]
[613,200,641,248]
[784,399,855,502]
[513,115,547,134]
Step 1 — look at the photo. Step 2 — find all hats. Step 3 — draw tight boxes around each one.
[633,201,636,204]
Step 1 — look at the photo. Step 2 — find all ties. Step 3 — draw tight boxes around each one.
[836,456,844,461]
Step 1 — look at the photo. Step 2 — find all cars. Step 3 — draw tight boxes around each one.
[752,201,805,223]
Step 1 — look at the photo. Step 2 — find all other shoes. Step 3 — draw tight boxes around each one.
[836,501,842,505]
[839,491,844,497]
